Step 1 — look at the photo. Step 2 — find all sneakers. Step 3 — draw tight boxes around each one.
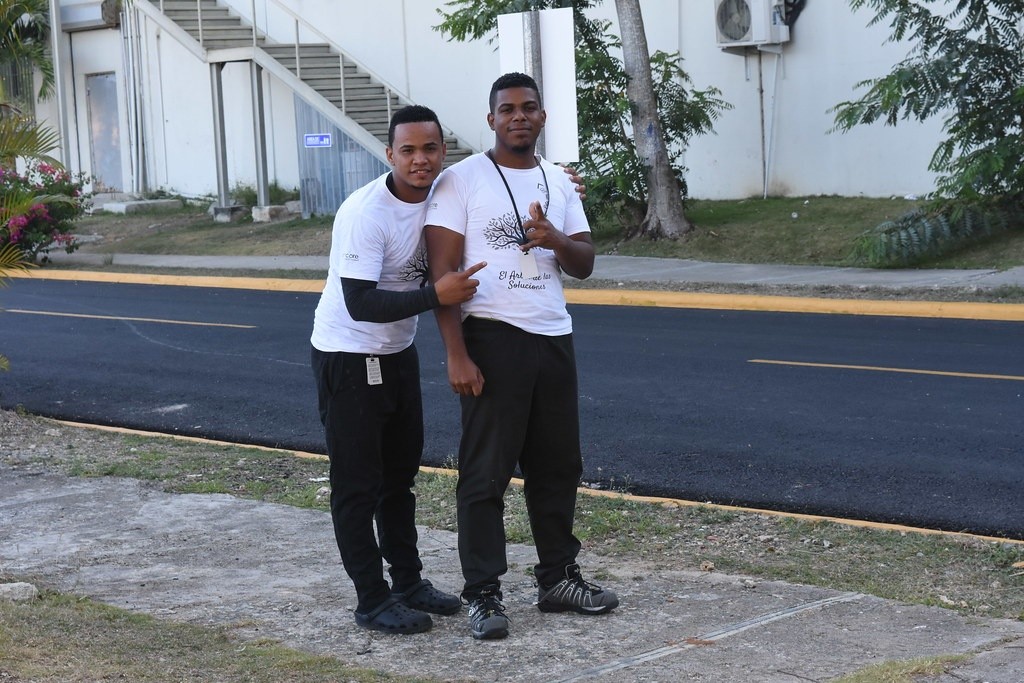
[534,563,619,614]
[460,584,511,639]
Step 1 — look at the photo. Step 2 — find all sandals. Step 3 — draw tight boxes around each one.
[354,597,433,634]
[391,579,462,615]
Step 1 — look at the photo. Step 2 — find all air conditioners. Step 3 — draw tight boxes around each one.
[715,0,790,47]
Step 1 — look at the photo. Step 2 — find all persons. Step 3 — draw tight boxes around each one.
[311,104,586,637]
[423,71,620,641]
